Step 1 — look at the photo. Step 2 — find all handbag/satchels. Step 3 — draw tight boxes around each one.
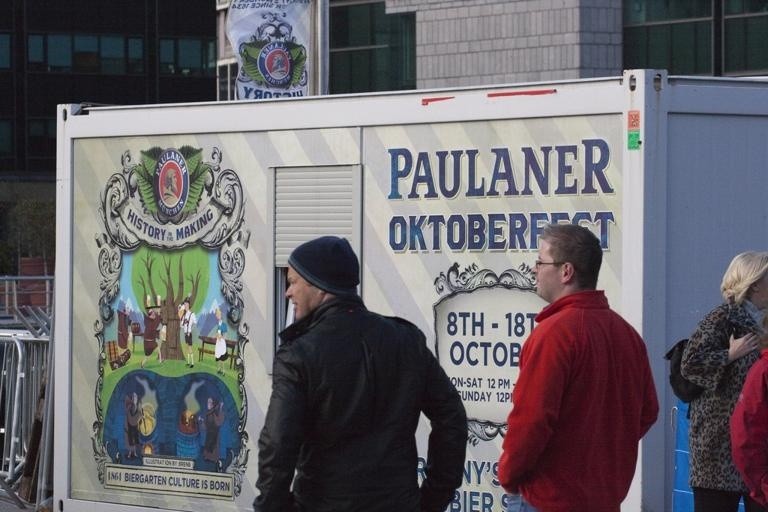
[664,339,704,404]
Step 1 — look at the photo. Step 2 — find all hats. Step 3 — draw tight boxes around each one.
[288,236,360,296]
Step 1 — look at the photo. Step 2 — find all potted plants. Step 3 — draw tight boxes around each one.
[0,231,15,305]
[12,197,56,284]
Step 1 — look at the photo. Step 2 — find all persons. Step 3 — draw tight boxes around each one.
[729,315,768,511]
[253,235,468,512]
[680,252,767,510]
[498,225,658,511]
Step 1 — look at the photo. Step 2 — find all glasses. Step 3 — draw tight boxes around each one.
[535,260,565,268]
